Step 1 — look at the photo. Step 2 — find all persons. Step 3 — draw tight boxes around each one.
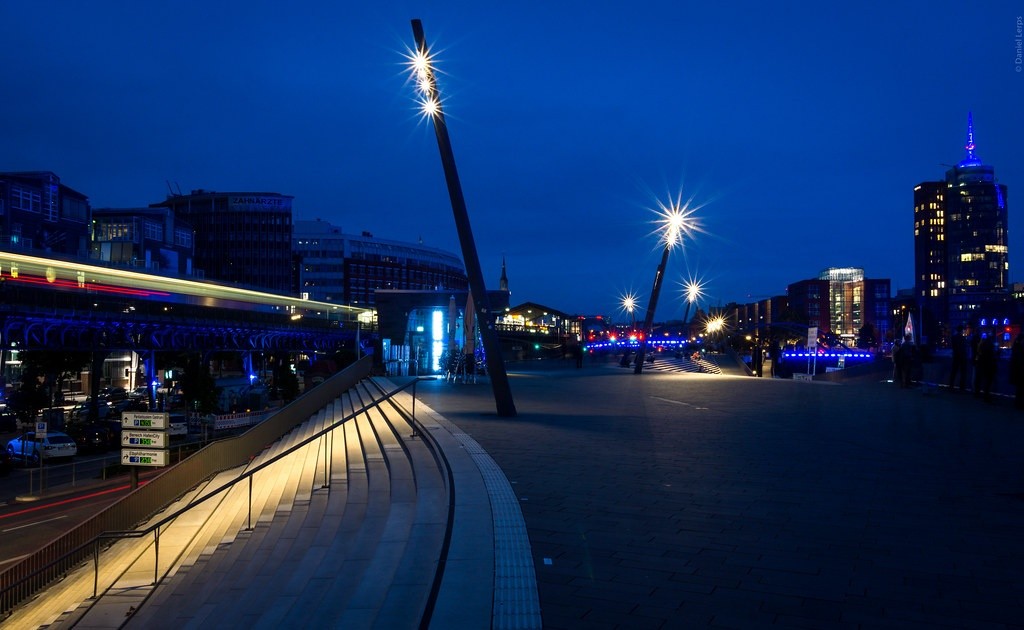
[576,345,583,369]
[1009,323,1024,411]
[693,351,701,360]
[948,324,1003,405]
[892,333,919,390]
[619,352,655,368]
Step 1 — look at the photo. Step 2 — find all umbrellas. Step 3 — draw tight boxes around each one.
[465,289,475,381]
[448,296,457,373]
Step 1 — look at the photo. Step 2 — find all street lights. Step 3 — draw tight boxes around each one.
[632,204,698,378]
[621,295,638,330]
[680,283,701,334]
[404,21,519,423]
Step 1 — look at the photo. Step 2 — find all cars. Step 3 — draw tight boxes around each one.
[60,385,148,454]
[0,405,18,433]
[0,443,14,479]
[5,428,78,467]
[168,412,188,439]
